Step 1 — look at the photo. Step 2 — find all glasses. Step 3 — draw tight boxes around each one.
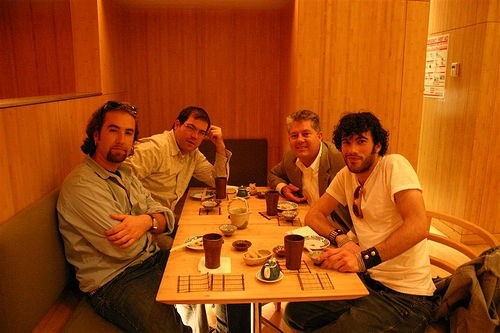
[352,186,363,218]
[101,102,137,116]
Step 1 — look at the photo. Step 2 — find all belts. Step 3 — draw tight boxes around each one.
[363,273,410,296]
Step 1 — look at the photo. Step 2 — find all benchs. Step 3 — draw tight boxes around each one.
[0,180,126,333]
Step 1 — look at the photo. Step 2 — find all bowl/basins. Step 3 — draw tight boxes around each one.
[218,224,237,237]
[282,210,297,221]
[307,251,324,265]
[232,240,252,250]
[244,249,273,266]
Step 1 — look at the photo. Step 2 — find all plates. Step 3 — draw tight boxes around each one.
[273,245,286,256]
[301,235,331,250]
[276,201,298,210]
[255,269,284,282]
[191,190,216,199]
[184,234,205,251]
[201,200,221,207]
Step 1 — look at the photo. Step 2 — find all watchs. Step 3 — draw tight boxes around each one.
[147,213,159,231]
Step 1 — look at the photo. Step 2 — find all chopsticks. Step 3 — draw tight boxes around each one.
[169,237,203,252]
[201,187,207,201]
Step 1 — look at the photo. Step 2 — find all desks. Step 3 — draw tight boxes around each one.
[157,186,371,333]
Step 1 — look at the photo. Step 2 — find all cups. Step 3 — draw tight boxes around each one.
[264,191,279,216]
[202,233,224,269]
[283,234,304,270]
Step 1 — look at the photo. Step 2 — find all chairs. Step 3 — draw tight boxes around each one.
[417,211,500,332]
[191,137,270,187]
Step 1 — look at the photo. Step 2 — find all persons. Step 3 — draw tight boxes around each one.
[57,101,193,333]
[283,112,436,333]
[268,109,352,234]
[124,107,232,333]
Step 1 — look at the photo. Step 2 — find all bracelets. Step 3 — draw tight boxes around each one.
[338,237,352,248]
[329,228,345,244]
[361,246,382,270]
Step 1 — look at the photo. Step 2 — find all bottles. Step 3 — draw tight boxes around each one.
[238,186,248,197]
[261,259,281,280]
[215,177,227,199]
